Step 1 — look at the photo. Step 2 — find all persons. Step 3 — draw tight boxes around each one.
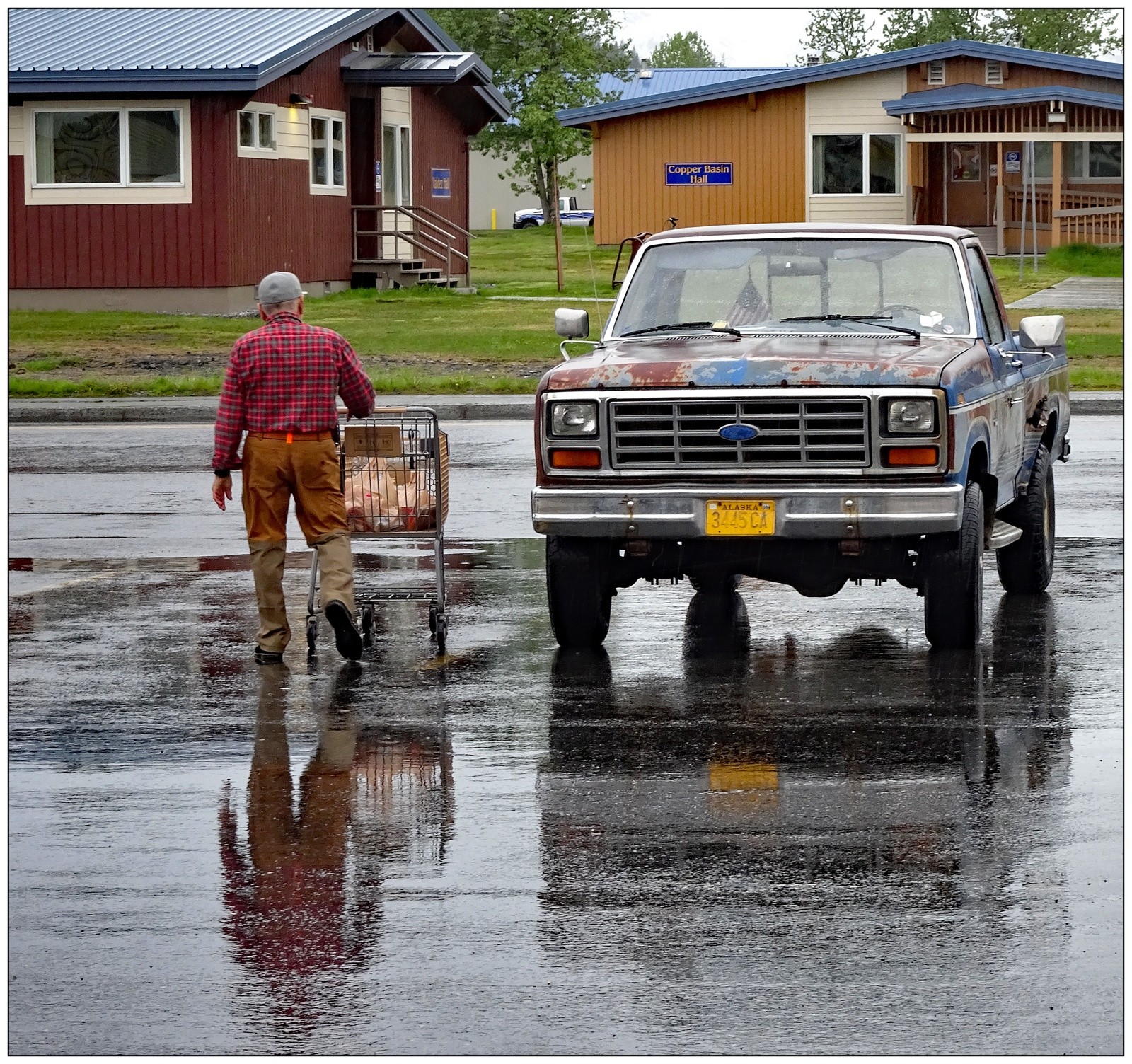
[212,271,376,665]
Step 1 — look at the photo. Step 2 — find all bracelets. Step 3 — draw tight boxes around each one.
[214,469,230,478]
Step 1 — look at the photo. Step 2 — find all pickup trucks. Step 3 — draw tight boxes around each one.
[513,196,594,230]
[531,220,1072,652]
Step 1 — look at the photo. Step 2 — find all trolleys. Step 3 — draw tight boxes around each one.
[306,407,451,652]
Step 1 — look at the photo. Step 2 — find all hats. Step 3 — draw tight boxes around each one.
[259,270,308,305]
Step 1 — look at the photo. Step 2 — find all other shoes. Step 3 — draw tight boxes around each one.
[253,645,283,664]
[325,600,363,661]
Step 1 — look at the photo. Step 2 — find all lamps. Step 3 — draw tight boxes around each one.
[1048,112,1068,124]
[289,94,307,105]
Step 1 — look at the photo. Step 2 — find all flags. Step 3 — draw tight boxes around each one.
[726,279,773,327]
